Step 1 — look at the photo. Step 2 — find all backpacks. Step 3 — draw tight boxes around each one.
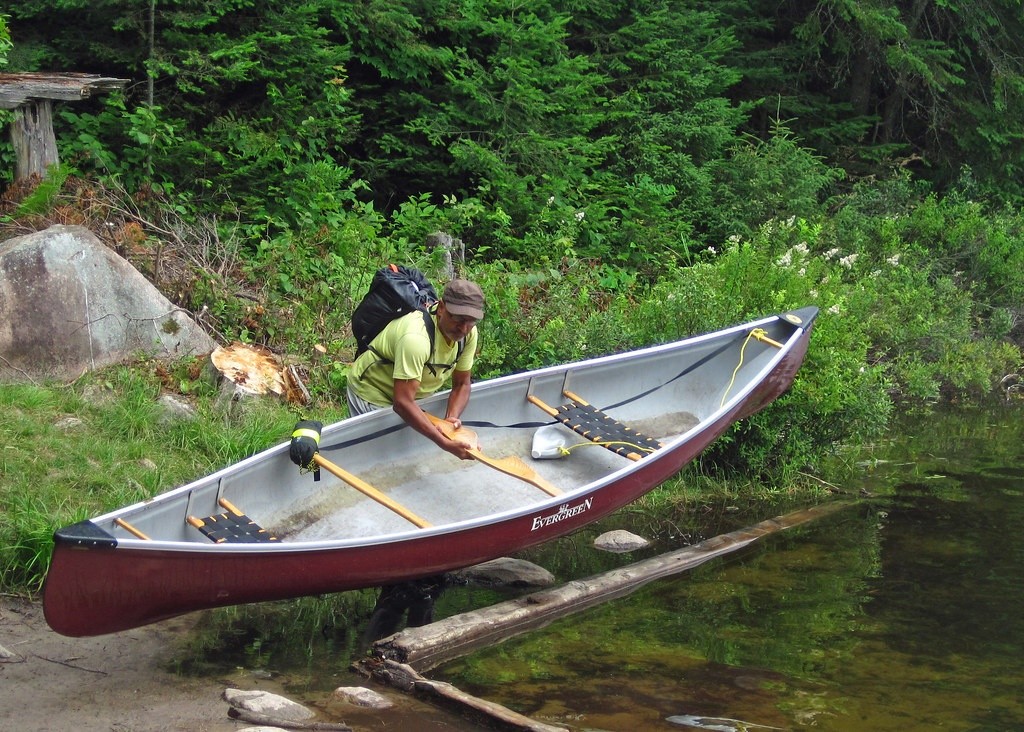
[351,265,466,380]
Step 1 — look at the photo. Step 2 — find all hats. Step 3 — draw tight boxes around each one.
[442,279,483,320]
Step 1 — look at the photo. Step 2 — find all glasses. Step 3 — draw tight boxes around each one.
[447,310,480,327]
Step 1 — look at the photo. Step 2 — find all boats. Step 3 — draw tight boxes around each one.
[41,302,824,640]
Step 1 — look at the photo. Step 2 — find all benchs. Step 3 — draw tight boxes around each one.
[186,499,284,544]
[528,389,668,462]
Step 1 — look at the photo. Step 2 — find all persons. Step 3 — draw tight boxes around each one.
[346,280,485,461]
